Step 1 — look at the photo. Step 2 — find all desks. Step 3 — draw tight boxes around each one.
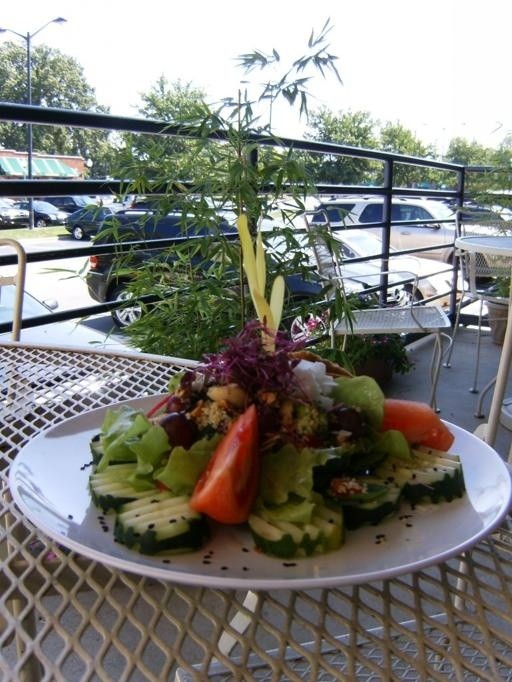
[0,344,512,682]
[443,235,512,393]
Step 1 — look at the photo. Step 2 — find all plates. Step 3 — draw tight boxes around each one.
[7,394,512,593]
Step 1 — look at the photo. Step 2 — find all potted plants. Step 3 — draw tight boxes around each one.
[485,277,512,345]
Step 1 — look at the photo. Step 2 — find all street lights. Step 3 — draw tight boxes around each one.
[0,16,68,229]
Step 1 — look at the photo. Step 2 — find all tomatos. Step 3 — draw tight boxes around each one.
[380,400,455,452]
[190,404,258,526]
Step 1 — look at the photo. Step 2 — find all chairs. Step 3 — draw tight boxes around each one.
[304,209,453,413]
